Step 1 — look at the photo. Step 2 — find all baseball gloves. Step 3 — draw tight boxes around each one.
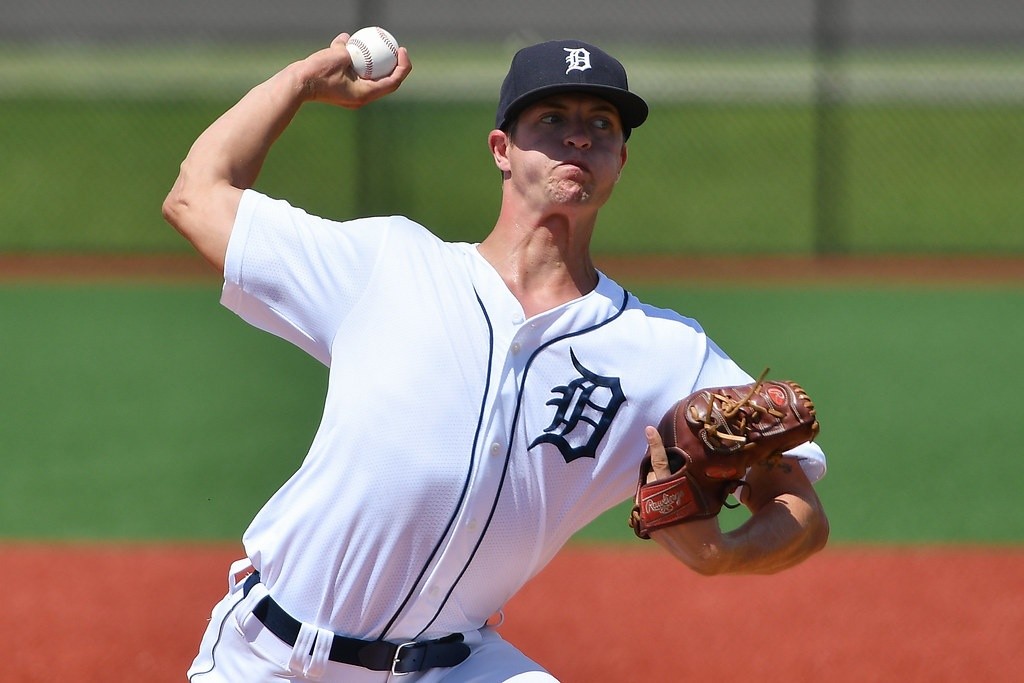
[620,373,818,540]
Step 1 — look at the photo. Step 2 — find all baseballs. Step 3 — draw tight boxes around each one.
[339,21,404,85]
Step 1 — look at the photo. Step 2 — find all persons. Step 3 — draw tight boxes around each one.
[161,33,830,682]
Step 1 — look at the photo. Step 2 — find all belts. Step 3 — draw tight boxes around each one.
[243,570,488,675]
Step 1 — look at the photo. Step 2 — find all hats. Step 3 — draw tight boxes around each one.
[498,39,649,134]
